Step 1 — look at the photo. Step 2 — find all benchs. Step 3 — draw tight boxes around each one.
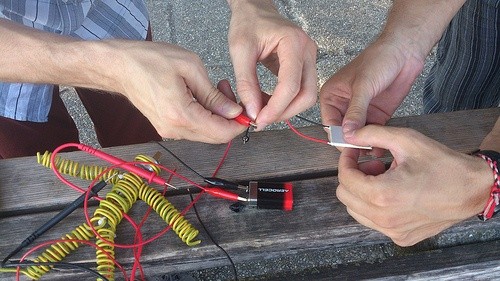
[0,108,500,281]
